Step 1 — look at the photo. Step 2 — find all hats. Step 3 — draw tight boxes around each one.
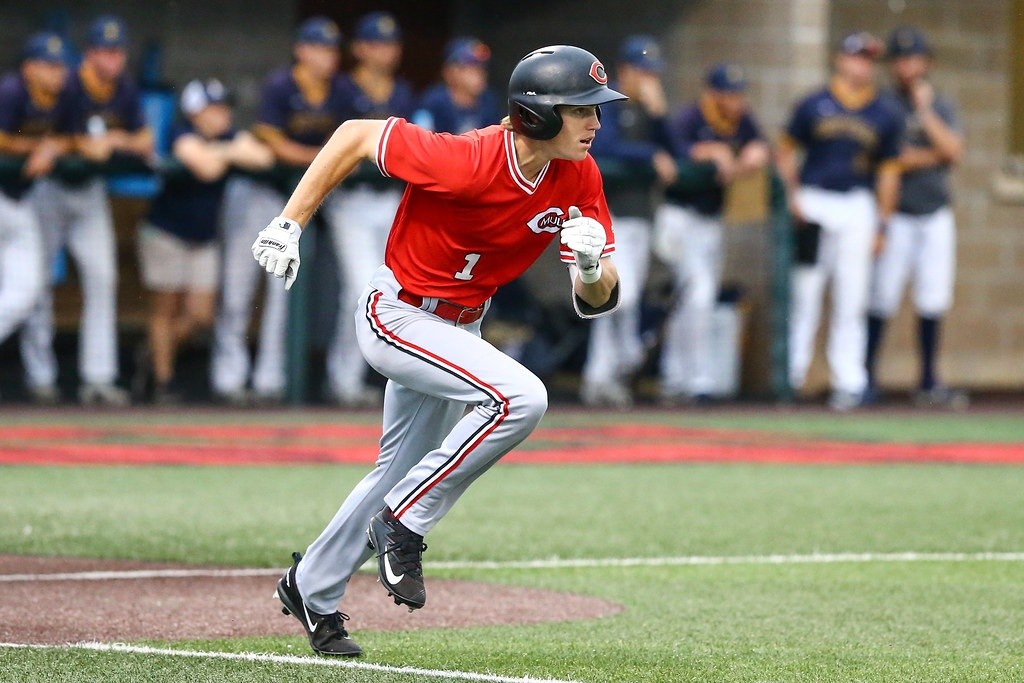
[17,32,83,66]
[182,75,231,105]
[707,61,748,91]
[295,14,342,44]
[839,28,883,58]
[442,35,494,68]
[353,8,404,42]
[84,13,132,49]
[888,25,931,55]
[617,33,668,73]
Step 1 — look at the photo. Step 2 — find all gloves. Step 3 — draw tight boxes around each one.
[252,216,304,291]
[560,205,607,284]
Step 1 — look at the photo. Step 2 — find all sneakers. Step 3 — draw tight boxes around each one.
[365,504,428,613]
[276,551,364,657]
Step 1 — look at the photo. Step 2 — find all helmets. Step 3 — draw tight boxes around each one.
[507,44,629,141]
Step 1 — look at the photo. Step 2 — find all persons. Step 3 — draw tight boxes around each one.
[0,11,962,407]
[252,44,631,657]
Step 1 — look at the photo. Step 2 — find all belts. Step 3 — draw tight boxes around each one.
[397,287,484,326]
